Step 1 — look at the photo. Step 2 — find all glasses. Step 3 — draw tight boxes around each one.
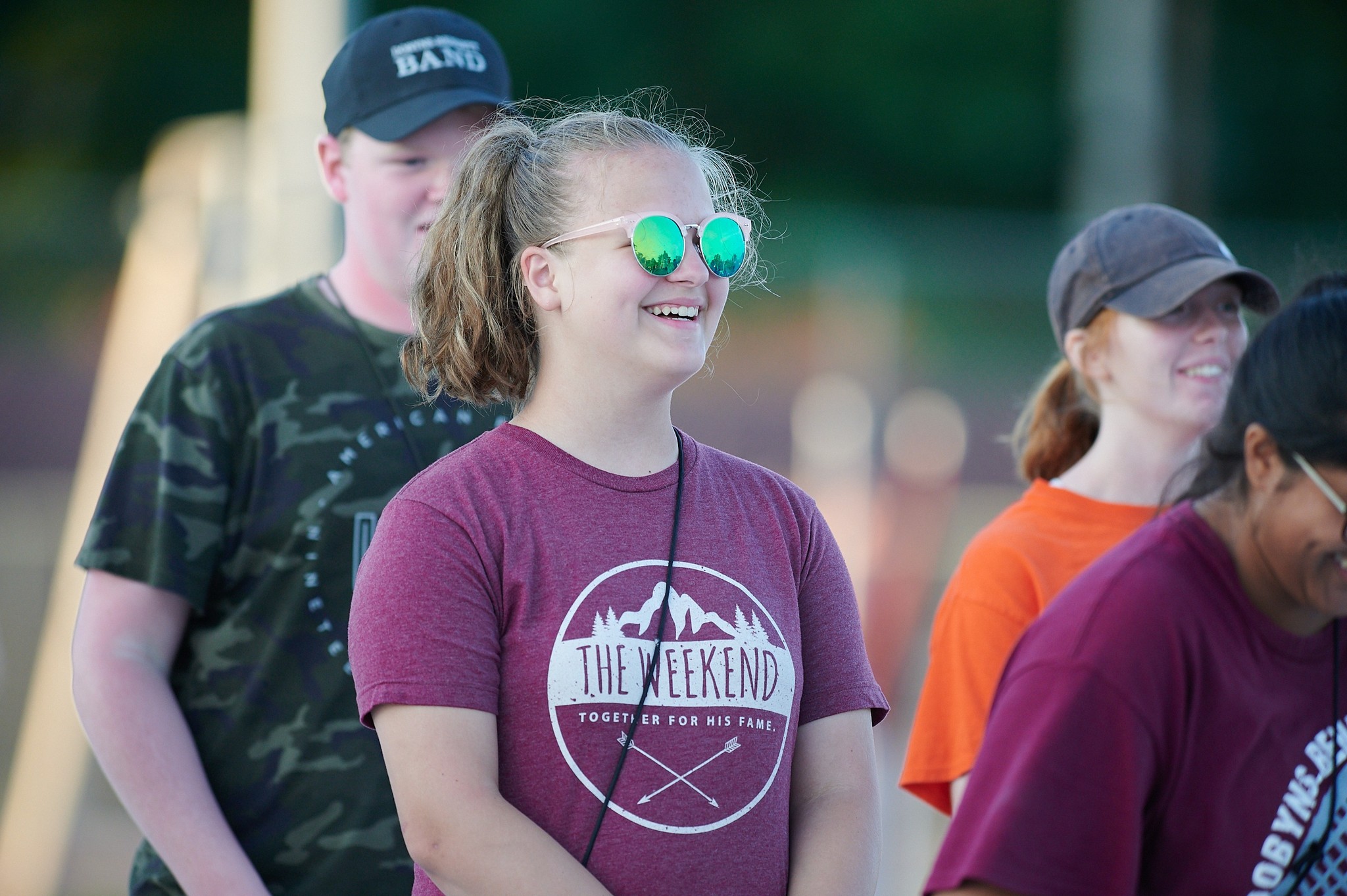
[1291,451,1347,543]
[540,210,752,277]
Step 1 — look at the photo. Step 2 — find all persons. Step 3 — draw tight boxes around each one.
[901,204,1283,824]
[71,6,521,895]
[922,274,1347,896]
[348,106,888,895]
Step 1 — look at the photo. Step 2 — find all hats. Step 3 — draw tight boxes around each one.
[1046,204,1280,358]
[322,7,515,142]
